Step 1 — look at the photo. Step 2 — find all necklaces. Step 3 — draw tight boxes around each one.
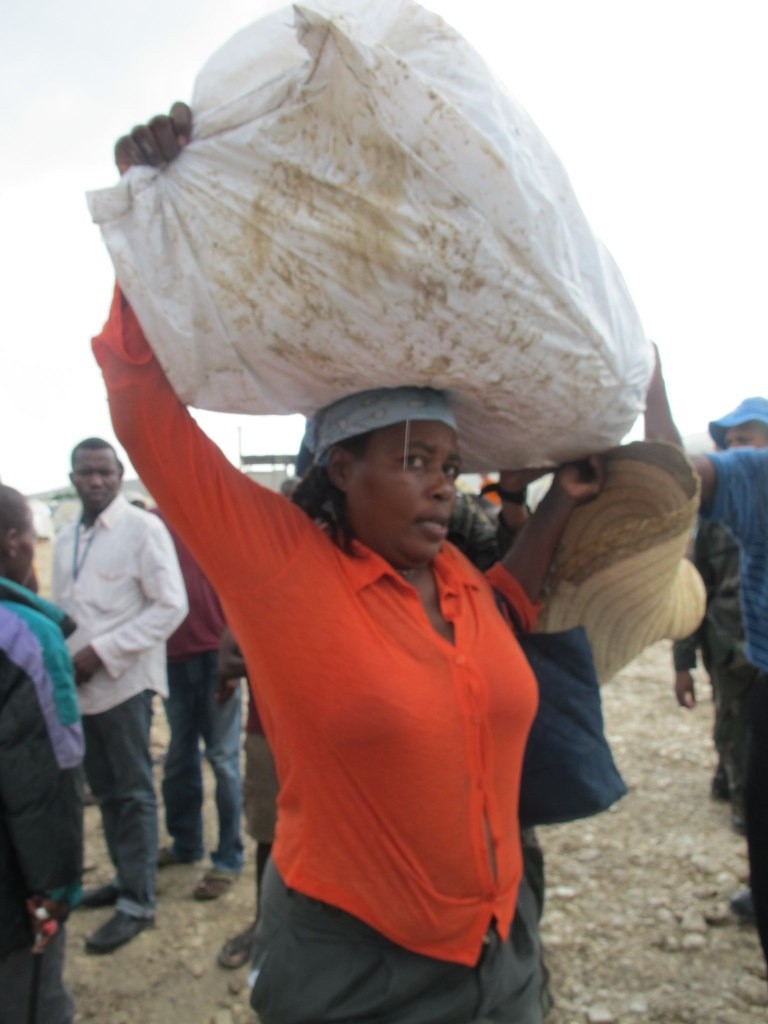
[395,567,419,575]
[73,525,93,581]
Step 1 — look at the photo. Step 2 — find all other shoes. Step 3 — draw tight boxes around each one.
[217,923,253,969]
[192,868,240,899]
[85,910,154,953]
[730,806,747,832]
[731,888,756,916]
[158,840,203,866]
[710,764,729,800]
[80,882,119,907]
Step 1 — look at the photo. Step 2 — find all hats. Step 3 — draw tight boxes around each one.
[708,397,768,451]
[538,442,706,688]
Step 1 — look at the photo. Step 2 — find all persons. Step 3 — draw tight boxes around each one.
[0,101,768,1024]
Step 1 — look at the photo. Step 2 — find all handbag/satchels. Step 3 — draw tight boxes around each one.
[494,586,628,829]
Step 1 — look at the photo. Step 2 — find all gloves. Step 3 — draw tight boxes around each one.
[27,888,72,953]
